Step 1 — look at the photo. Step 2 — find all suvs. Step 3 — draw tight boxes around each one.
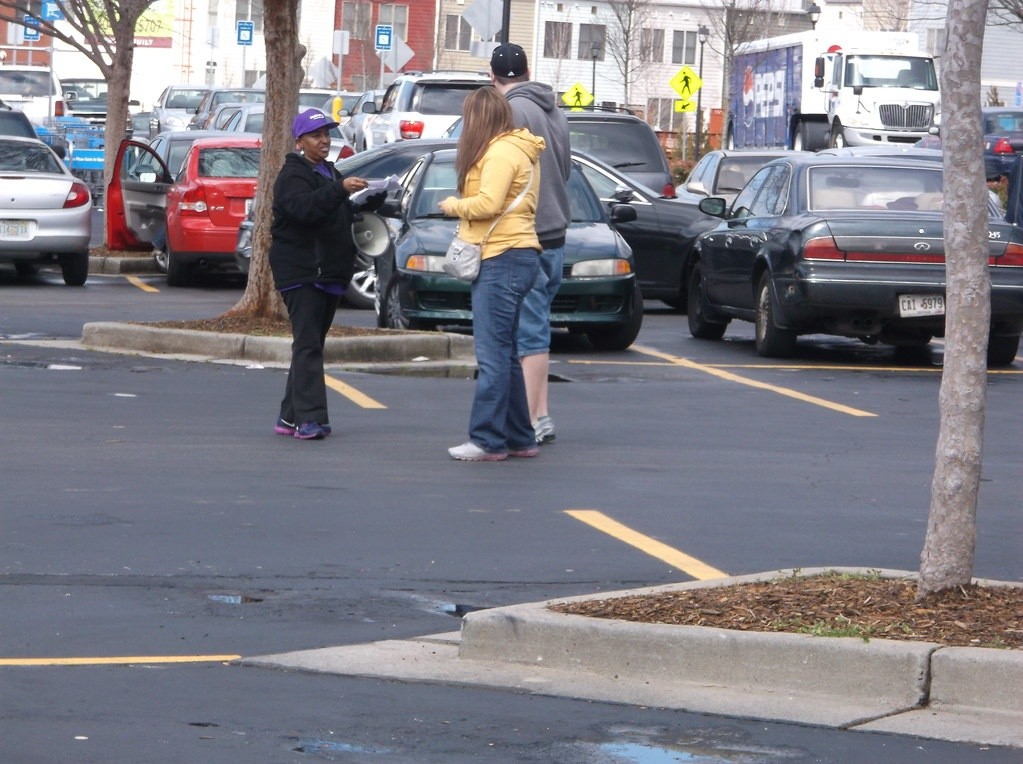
[354,69,494,152]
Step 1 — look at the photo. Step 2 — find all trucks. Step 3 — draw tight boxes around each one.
[726,27,944,153]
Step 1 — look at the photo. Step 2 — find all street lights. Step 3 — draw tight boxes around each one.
[589,41,600,112]
[692,25,710,162]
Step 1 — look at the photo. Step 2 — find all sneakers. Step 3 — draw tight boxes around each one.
[507,443,538,456]
[275,416,331,435]
[295,421,325,439]
[448,442,508,461]
[533,417,555,444]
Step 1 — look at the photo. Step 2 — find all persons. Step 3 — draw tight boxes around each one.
[267,107,388,441]
[437,85,546,461]
[490,43,572,443]
[151,153,215,273]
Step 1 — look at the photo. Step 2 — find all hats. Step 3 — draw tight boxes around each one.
[490,43,527,78]
[291,109,339,139]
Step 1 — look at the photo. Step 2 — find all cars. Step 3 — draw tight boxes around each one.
[105,138,302,288]
[676,148,808,210]
[682,151,1023,369]
[818,107,1022,224]
[0,135,94,287]
[369,149,645,355]
[129,130,260,182]
[335,137,731,314]
[0,65,674,204]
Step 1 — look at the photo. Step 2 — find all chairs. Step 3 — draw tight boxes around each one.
[210,160,232,176]
[914,192,944,211]
[811,190,854,210]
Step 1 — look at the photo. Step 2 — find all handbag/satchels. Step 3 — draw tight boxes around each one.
[442,237,481,281]
